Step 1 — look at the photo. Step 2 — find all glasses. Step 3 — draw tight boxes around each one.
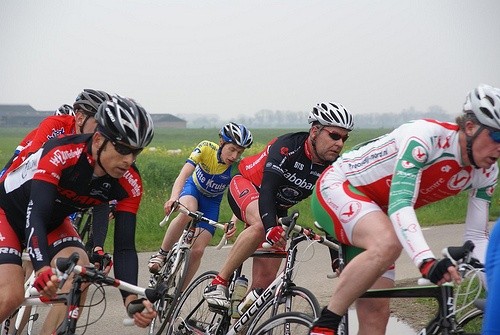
[484,126,500,143]
[101,133,143,157]
[322,128,349,143]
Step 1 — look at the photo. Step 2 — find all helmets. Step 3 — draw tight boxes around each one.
[463,84,500,130]
[218,121,254,149]
[73,89,112,115]
[308,102,355,132]
[54,104,76,116]
[94,95,154,150]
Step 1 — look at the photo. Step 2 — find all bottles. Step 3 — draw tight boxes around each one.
[231,275,248,318]
[237,288,264,315]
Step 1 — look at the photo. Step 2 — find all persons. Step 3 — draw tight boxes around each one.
[307,84,499,335]
[204,101,355,316]
[0,89,156,335]
[148,122,253,322]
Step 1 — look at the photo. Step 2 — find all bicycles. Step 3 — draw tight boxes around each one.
[147,199,234,335]
[335,238,490,335]
[164,208,345,335]
[1,250,158,335]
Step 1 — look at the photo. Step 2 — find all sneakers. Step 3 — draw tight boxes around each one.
[148,254,167,273]
[164,295,179,322]
[202,282,231,310]
